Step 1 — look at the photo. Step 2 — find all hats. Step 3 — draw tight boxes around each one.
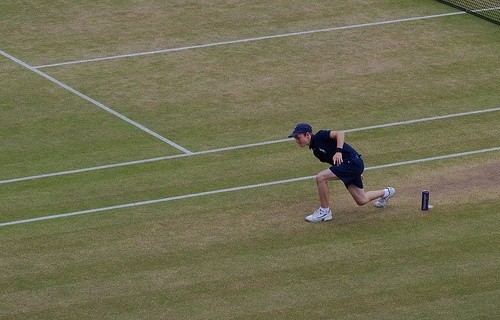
[288,122,312,138]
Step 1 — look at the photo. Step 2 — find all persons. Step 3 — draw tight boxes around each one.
[288,123,395,223]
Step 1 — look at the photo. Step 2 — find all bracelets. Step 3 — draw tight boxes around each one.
[336,148,343,153]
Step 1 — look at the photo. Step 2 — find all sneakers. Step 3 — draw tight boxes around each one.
[375,187,395,208]
[305,207,332,222]
